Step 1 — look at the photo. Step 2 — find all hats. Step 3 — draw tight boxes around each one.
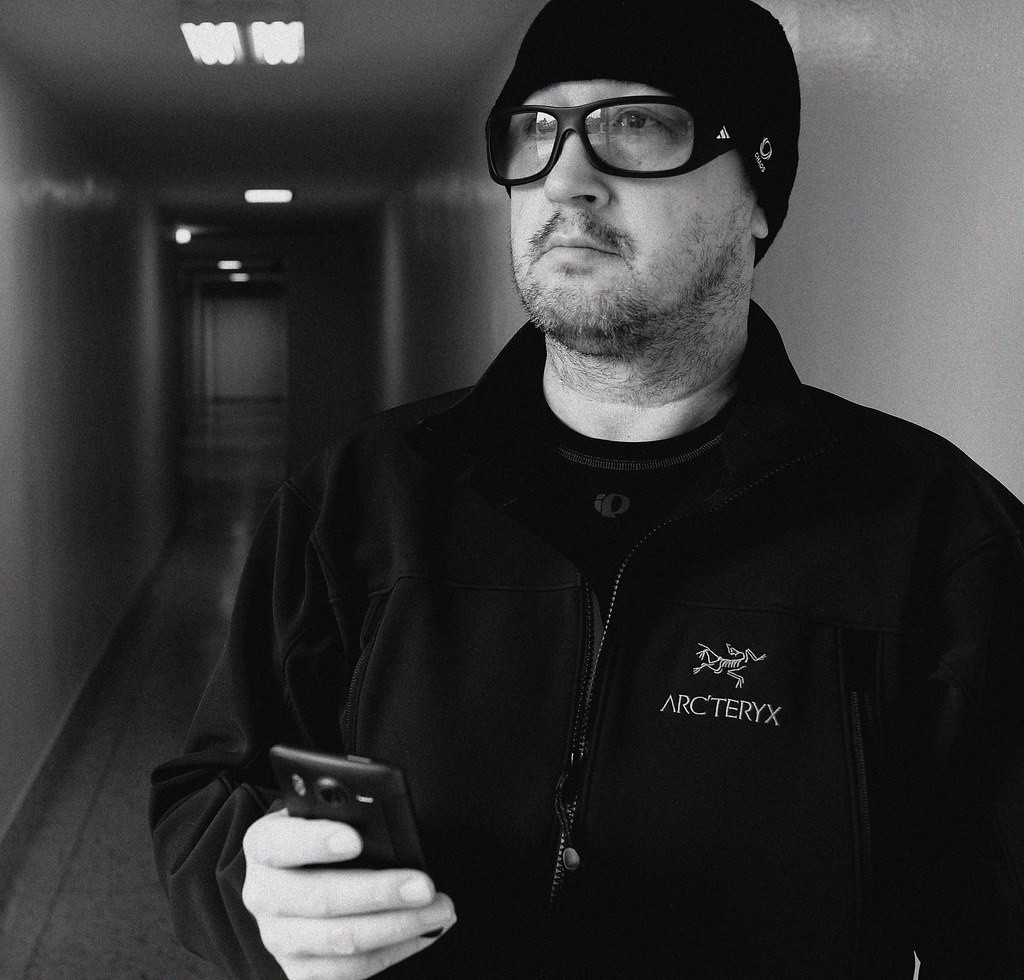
[488,0,802,267]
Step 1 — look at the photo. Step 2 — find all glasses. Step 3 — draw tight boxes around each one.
[486,95,737,198]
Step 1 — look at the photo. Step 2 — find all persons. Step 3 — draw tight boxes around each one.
[149,0,1024,980]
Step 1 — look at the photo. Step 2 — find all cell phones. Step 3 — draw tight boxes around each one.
[271,744,444,938]
